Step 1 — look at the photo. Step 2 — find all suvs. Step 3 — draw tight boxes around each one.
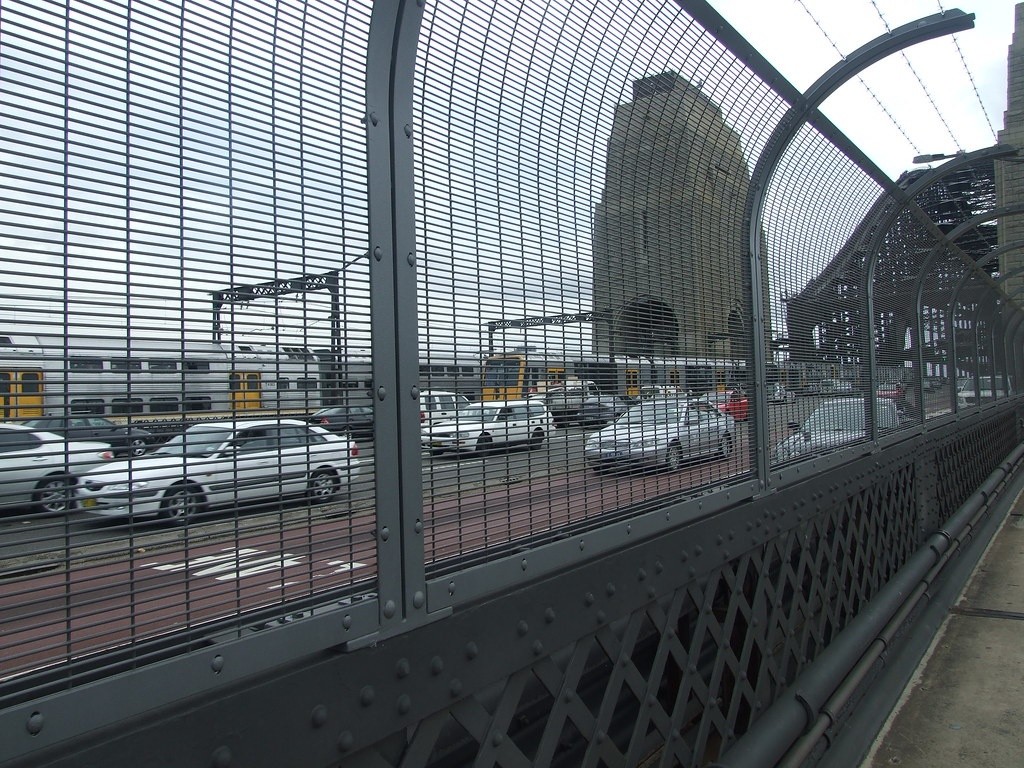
[420,390,472,428]
[641,385,684,399]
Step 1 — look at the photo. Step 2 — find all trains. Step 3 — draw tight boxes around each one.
[481,354,913,419]
[2,344,501,468]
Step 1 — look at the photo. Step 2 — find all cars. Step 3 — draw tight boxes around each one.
[767,384,797,404]
[419,401,557,459]
[0,425,116,517]
[584,399,736,474]
[956,376,1012,409]
[835,382,854,396]
[578,396,636,428]
[22,418,153,457]
[876,383,906,400]
[770,398,917,466]
[923,379,942,393]
[309,405,373,440]
[75,421,362,526]
[698,392,748,422]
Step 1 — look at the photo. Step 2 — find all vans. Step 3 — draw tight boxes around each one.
[818,379,839,395]
